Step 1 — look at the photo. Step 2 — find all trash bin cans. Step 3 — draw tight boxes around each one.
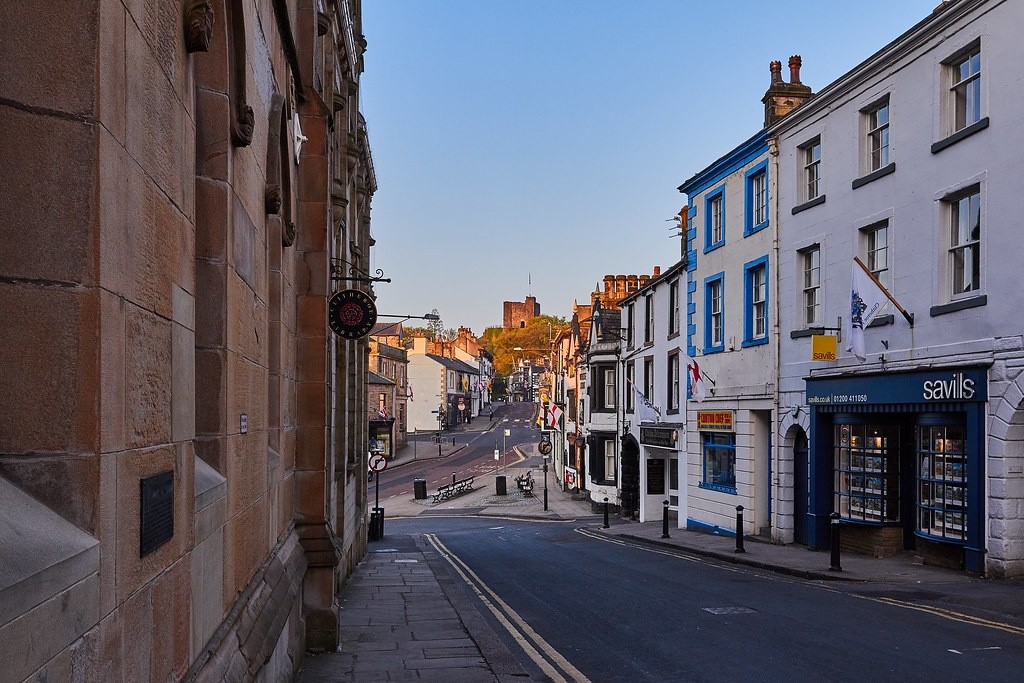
[496,475,507,495]
[413,479,427,499]
[369,507,385,540]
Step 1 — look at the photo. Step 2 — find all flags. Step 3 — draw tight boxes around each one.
[851,260,889,331]
[378,408,389,425]
[409,387,413,401]
[487,380,492,393]
[547,401,564,432]
[540,399,545,420]
[680,351,705,403]
[462,377,469,392]
[632,385,660,425]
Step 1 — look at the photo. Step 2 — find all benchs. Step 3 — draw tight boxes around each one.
[515,471,531,488]
[444,480,464,497]
[458,475,475,494]
[520,475,531,493]
[523,479,535,498]
[430,484,451,503]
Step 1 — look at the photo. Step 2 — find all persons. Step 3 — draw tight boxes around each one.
[370,437,379,449]
[489,409,492,421]
[466,410,472,424]
